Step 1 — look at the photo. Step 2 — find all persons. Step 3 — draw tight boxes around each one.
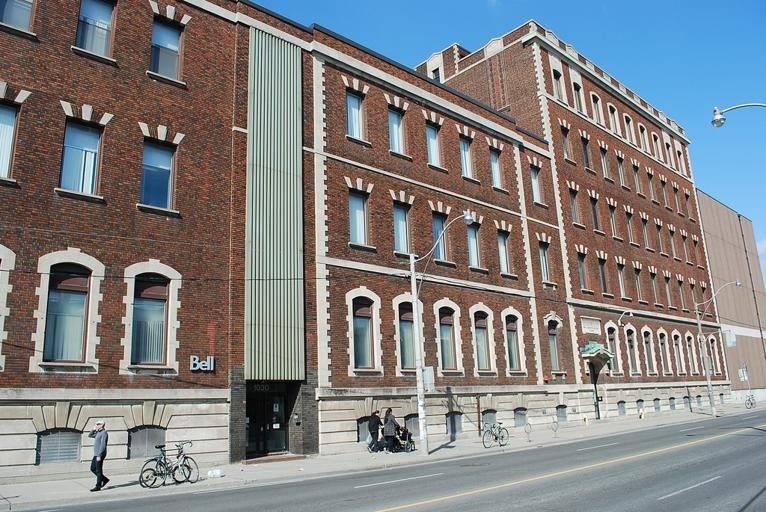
[89,419,110,491]
[382,408,399,454]
[367,410,384,453]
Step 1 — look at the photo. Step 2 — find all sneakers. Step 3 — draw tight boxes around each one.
[90,479,109,491]
[368,446,393,454]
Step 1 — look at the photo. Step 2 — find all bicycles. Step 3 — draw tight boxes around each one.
[482,422,509,448]
[745,394,756,409]
[139,440,199,488]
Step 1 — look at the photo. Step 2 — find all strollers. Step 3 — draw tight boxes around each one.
[380,427,415,453]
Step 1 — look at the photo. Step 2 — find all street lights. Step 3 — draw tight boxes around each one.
[712,103,766,127]
[407,210,473,456]
[691,279,741,415]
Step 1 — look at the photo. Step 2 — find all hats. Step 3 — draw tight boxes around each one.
[94,420,104,426]
